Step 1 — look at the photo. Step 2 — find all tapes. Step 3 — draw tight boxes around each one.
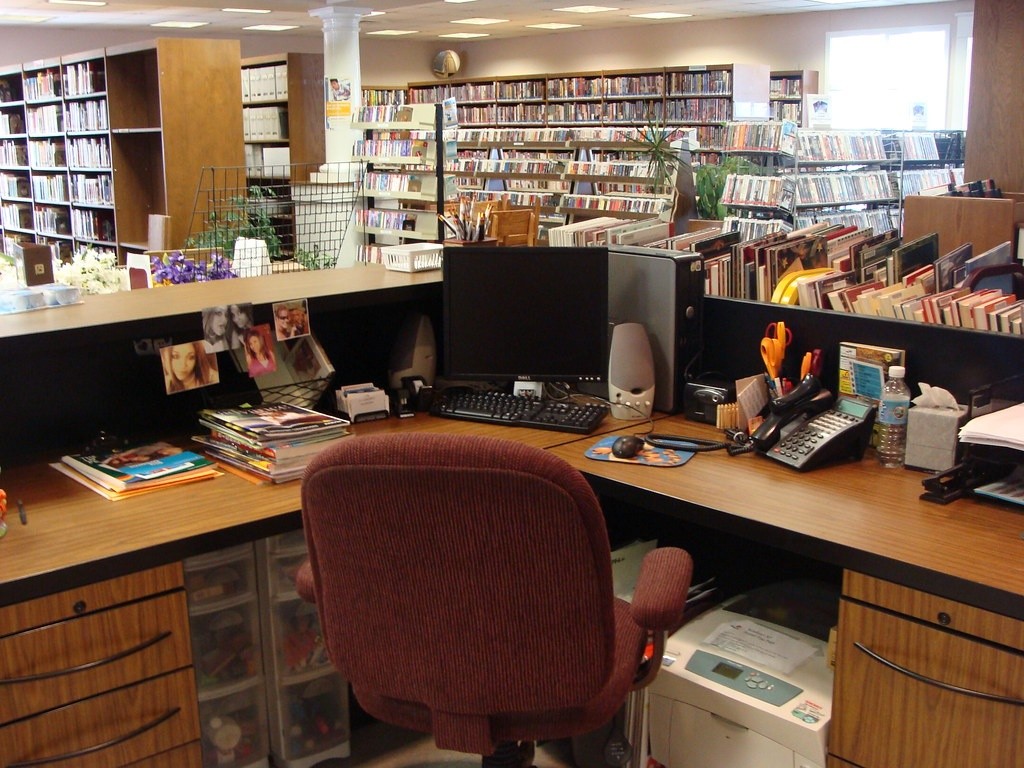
[411,380,423,393]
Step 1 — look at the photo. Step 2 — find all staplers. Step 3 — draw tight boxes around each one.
[392,388,414,418]
[919,458,989,504]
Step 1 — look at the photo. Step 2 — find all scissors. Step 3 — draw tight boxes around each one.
[801,350,823,378]
[761,321,793,388]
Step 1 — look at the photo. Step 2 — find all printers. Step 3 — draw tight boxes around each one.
[646,575,834,768]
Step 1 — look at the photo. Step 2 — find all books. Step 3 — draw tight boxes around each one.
[0,135,113,206]
[836,342,905,448]
[722,119,797,241]
[352,105,436,160]
[410,70,730,123]
[794,129,965,240]
[49,440,225,500]
[0,202,117,266]
[544,213,1024,335]
[0,60,109,132]
[769,78,802,127]
[642,120,725,189]
[350,169,421,264]
[276,324,335,403]
[187,404,356,486]
[361,90,409,107]
[456,128,682,239]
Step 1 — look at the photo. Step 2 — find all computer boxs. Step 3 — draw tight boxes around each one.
[604,244,706,414]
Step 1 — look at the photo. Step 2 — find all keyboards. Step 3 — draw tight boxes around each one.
[432,390,608,434]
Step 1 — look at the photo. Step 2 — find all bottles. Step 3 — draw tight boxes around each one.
[876,366,911,468]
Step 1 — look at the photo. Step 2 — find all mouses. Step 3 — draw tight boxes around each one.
[612,436,644,459]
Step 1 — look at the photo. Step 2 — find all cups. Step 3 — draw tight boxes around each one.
[0,283,79,312]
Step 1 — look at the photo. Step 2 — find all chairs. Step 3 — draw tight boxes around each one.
[296,430,693,767]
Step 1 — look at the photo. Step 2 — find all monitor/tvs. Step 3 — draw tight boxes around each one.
[441,246,610,400]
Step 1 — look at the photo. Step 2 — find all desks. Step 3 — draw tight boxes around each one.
[2,377,1024,768]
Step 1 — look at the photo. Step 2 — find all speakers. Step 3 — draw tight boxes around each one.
[608,323,655,421]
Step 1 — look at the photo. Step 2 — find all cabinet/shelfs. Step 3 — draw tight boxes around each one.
[239,52,326,185]
[360,63,968,241]
[0,36,246,269]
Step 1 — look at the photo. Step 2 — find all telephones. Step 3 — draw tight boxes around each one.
[751,388,876,472]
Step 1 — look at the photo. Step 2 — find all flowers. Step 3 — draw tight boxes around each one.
[52,242,131,296]
[149,248,238,286]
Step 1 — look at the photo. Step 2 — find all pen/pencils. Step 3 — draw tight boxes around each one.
[764,372,779,398]
[775,376,791,397]
[437,213,456,235]
[18,497,27,525]
[448,193,494,242]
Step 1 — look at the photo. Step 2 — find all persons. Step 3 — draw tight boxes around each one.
[202,302,254,353]
[330,79,351,100]
[278,304,309,337]
[254,408,332,427]
[244,329,278,378]
[164,343,219,392]
